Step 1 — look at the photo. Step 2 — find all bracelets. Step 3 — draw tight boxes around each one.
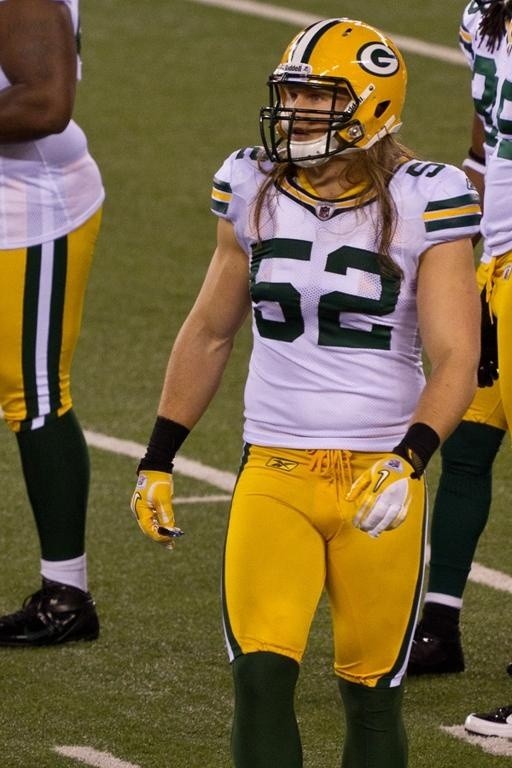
[393,424,440,473]
[143,416,190,464]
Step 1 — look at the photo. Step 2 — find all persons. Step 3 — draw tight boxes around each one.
[130,17,484,768]
[0,0,106,648]
[406,0,512,741]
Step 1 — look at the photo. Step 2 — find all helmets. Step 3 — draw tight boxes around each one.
[258,17,408,172]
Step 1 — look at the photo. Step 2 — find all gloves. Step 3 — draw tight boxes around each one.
[474,282,499,388]
[130,458,184,550]
[345,447,423,537]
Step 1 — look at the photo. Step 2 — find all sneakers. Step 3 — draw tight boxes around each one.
[408,621,466,676]
[465,706,512,740]
[0,576,100,648]
